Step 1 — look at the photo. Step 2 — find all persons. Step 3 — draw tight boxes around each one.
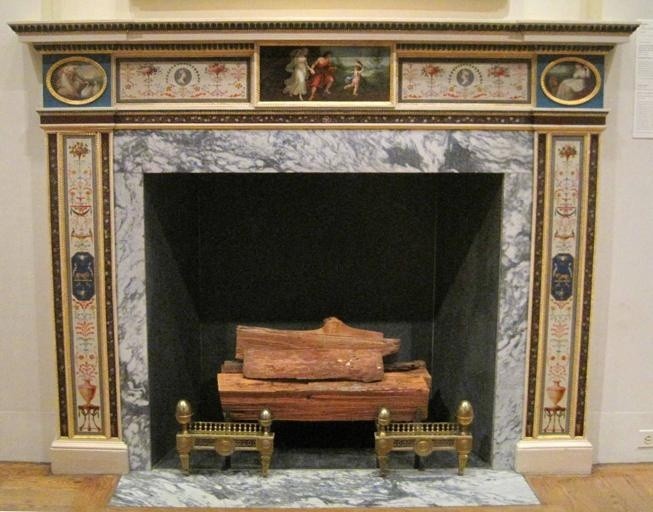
[344,61,364,97]
[58,61,92,101]
[555,63,590,99]
[308,51,336,101]
[282,48,315,100]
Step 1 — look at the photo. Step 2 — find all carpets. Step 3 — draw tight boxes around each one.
[108,441,543,510]
